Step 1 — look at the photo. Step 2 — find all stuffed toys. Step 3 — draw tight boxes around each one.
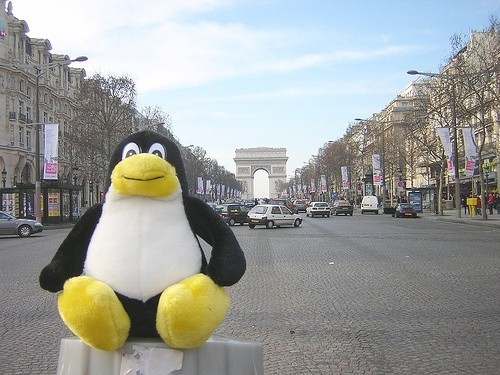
[39,128,247,350]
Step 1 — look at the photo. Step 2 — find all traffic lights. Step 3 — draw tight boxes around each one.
[89,180,93,192]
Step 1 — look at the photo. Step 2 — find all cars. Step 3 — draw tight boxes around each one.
[330,200,354,216]
[207,198,311,229]
[383,203,418,218]
[0,211,45,238]
[306,202,332,218]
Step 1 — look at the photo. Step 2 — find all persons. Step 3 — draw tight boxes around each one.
[460,193,481,215]
[385,194,389,199]
[349,194,362,207]
[373,195,383,206]
[392,195,408,203]
[485,191,500,215]
[255,198,270,206]
[443,193,456,208]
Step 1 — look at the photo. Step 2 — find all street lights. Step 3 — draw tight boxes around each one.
[329,140,352,199]
[397,168,402,203]
[330,182,334,192]
[1,166,7,186]
[483,163,490,194]
[355,118,387,205]
[407,70,462,218]
[72,162,78,185]
[96,179,99,203]
[35,56,89,223]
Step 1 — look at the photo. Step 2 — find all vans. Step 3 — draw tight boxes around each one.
[361,195,380,214]
